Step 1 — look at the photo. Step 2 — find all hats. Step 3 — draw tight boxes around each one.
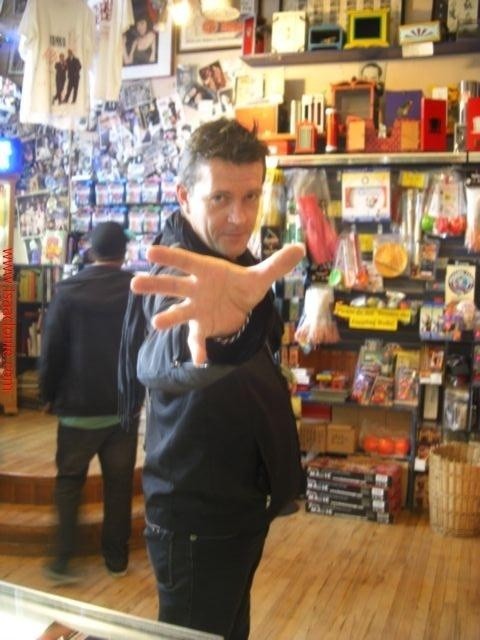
[90,222,130,256]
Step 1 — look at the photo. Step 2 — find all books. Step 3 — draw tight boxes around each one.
[17,373,41,400]
[15,268,61,303]
[17,309,41,355]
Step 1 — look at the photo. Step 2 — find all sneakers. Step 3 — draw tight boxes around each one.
[104,562,129,578]
[44,560,71,580]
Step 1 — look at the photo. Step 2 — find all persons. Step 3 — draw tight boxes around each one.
[62,50,82,104]
[52,54,67,104]
[362,63,385,131]
[130,118,308,640]
[38,221,144,578]
[119,16,157,66]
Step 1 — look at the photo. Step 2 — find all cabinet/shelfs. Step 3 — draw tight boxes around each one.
[14,263,80,413]
[250,151,480,512]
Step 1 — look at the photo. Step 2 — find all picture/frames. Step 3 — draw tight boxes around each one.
[123,1,174,80]
[178,1,243,54]
